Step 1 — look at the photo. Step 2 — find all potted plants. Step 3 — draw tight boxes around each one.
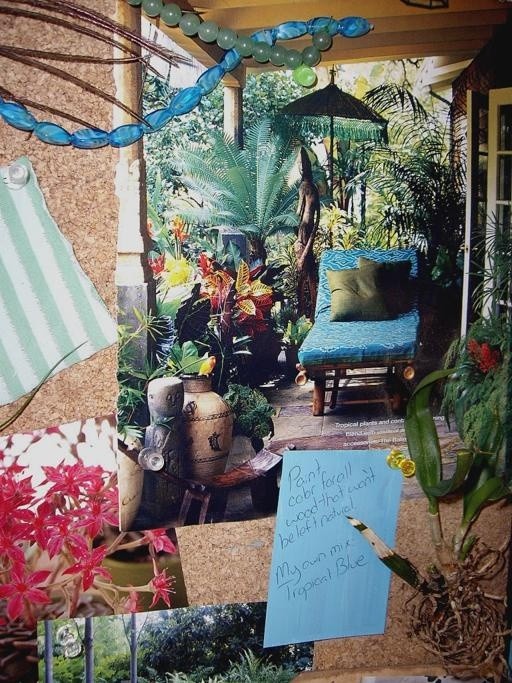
[118,303,167,533]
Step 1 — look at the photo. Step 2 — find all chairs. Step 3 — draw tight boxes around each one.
[298,250,420,416]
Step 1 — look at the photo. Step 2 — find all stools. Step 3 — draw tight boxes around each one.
[178,448,282,524]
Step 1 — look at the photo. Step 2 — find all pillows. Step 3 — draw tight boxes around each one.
[359,256,412,314]
[325,262,395,321]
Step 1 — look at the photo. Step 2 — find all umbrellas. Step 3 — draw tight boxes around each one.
[280,64,387,198]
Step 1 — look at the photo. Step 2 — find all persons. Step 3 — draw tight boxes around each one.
[295,146,319,324]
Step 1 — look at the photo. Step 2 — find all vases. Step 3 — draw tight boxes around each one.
[181,373,233,476]
